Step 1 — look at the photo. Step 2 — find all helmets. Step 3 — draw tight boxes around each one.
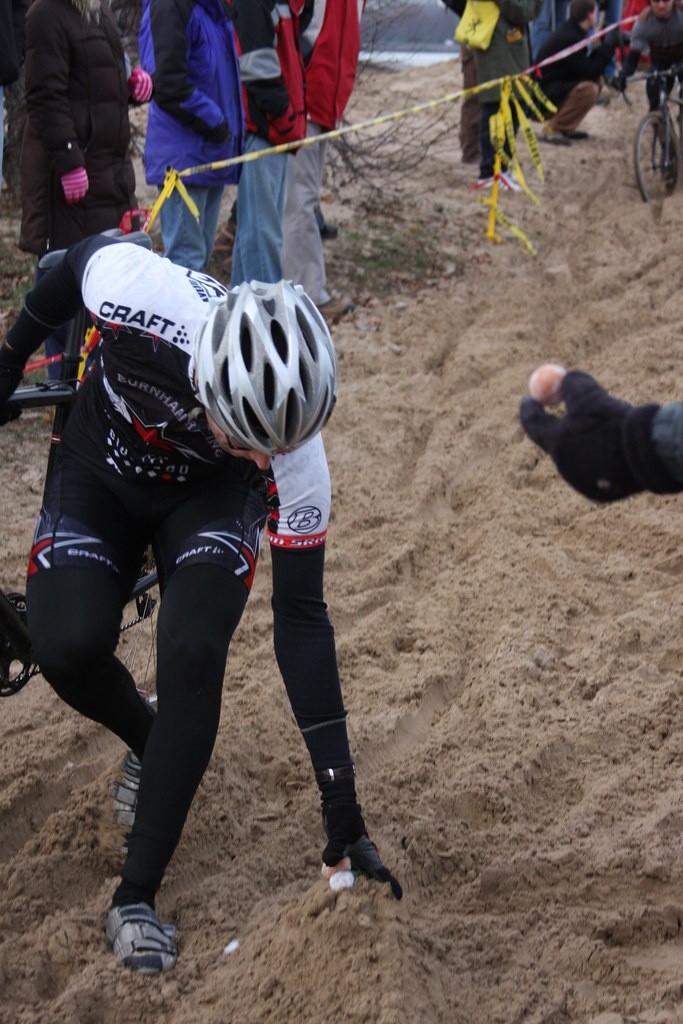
[191,279,336,459]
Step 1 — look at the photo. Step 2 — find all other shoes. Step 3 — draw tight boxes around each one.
[564,130,589,139]
[535,133,569,145]
[462,152,485,166]
[211,223,237,274]
[319,224,338,238]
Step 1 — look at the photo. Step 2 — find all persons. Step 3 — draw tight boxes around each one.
[2,0,366,383]
[0,231,403,977]
[443,0,682,192]
[521,369,683,504]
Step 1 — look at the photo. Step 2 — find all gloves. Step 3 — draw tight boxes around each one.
[318,778,402,900]
[0,341,22,426]
[129,68,152,103]
[61,166,89,203]
[610,74,626,91]
[519,372,683,502]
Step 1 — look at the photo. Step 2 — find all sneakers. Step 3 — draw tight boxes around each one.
[112,751,143,829]
[478,174,519,192]
[106,901,177,975]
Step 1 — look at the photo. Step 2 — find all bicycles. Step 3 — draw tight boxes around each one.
[625,65,683,202]
